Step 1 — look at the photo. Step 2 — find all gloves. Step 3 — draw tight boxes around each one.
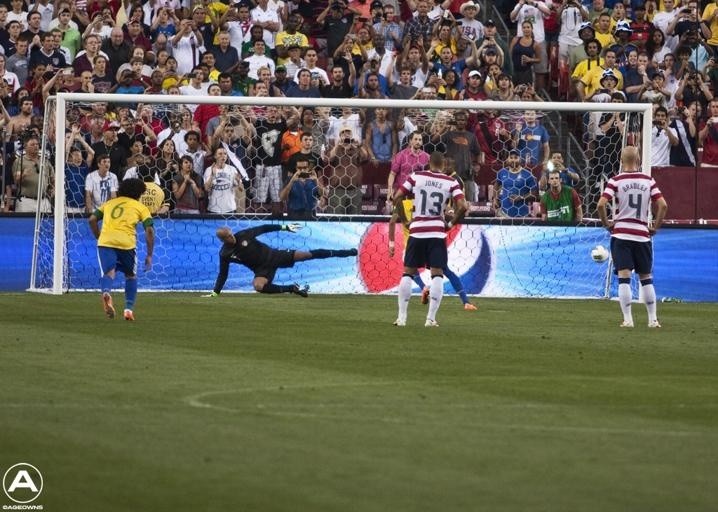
[286,223,302,232]
[201,292,217,298]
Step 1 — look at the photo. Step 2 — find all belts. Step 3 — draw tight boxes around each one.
[20,195,46,200]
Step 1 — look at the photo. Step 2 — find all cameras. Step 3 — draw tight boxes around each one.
[518,83,528,92]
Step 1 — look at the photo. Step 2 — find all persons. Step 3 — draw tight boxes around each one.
[597,145,668,328]
[1,2,717,221]
[89,177,155,320]
[200,223,357,299]
[388,194,476,310]
[392,153,467,328]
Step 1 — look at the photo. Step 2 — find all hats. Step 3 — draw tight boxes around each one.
[460,1,480,16]
[614,21,632,37]
[652,72,664,78]
[485,48,496,55]
[102,121,120,131]
[275,64,286,72]
[58,7,70,16]
[600,69,618,88]
[509,148,521,156]
[578,22,596,39]
[370,1,382,9]
[468,70,480,77]
[338,125,352,135]
[482,19,494,26]
[128,16,139,23]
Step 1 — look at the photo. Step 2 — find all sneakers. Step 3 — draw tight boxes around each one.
[424,320,439,327]
[103,292,115,319]
[292,284,307,297]
[647,321,660,328]
[463,304,477,310]
[393,318,407,326]
[340,248,358,257]
[619,322,633,328]
[123,311,133,321]
[421,287,430,305]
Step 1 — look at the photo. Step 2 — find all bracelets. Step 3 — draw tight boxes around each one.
[389,241,396,247]
[447,221,454,229]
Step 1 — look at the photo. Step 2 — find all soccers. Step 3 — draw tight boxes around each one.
[591,246,608,262]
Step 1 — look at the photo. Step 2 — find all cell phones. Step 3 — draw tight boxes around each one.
[63,70,71,74]
[227,104,234,112]
[187,73,196,77]
[600,88,608,93]
[297,172,309,178]
[456,20,462,25]
[681,9,690,13]
[677,106,684,111]
[343,138,353,143]
[382,12,387,21]
[652,120,661,125]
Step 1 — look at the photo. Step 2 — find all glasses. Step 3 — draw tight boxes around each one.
[34,162,40,174]
